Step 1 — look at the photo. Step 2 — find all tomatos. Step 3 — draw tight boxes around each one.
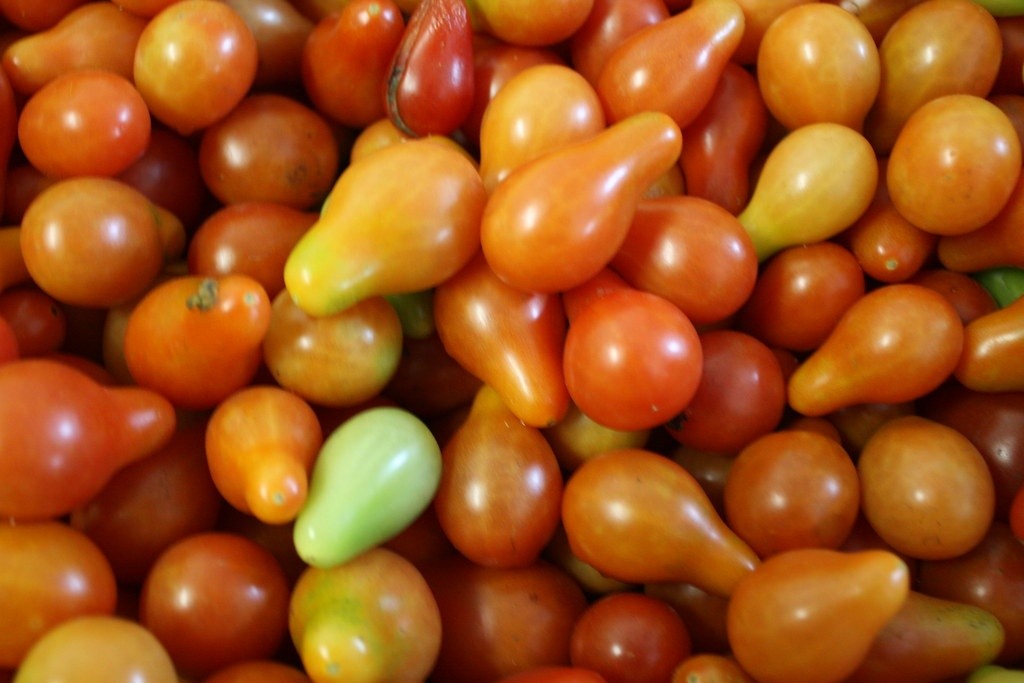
[0,0,1024,683]
